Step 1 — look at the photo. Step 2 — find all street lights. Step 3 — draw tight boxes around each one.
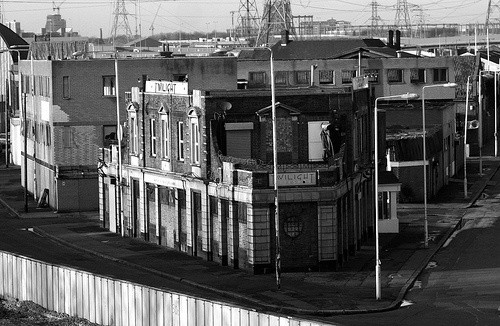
[212,46,282,291]
[421,84,458,250]
[464,73,494,200]
[71,49,123,236]
[373,92,421,300]
[0,49,29,214]
